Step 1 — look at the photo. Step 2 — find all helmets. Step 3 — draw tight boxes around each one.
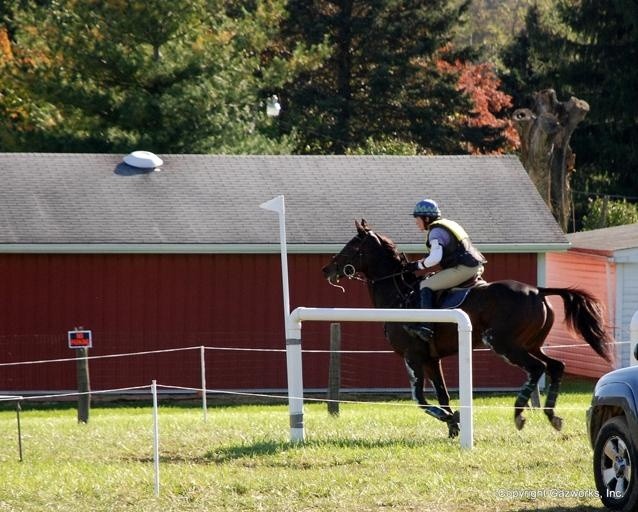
[409,199,441,217]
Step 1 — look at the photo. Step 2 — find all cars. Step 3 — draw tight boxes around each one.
[584,345,636,512]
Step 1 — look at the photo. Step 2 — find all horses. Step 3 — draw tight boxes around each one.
[319,217,617,439]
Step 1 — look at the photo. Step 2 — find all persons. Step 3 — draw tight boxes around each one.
[404,198,490,341]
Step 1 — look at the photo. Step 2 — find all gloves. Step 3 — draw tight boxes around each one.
[402,260,420,273]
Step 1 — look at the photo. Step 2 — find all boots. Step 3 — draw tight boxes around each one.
[402,287,434,341]
